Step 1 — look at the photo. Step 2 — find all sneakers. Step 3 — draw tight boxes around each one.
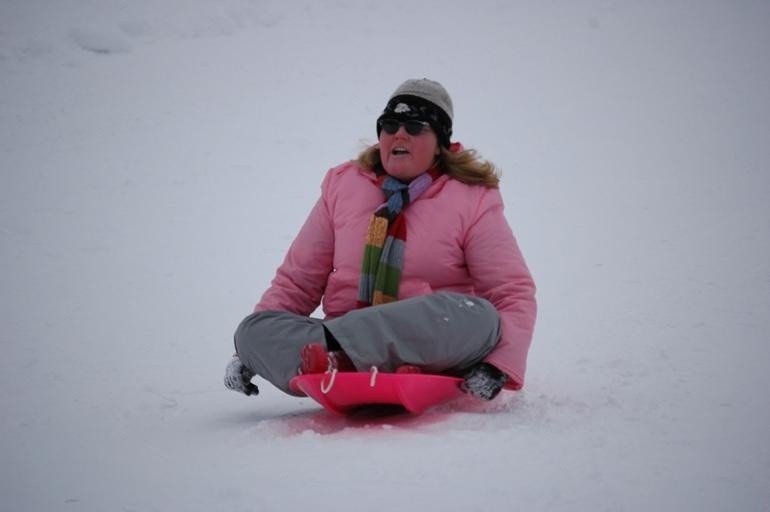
[299,343,344,375]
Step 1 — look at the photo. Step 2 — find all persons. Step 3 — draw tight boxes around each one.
[224,77,540,400]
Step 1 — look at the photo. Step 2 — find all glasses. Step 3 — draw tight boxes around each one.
[380,119,430,135]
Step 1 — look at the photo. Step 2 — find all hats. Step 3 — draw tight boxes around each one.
[377,79,454,149]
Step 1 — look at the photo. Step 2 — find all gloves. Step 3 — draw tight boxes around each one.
[224,353,259,396]
[458,363,508,400]
